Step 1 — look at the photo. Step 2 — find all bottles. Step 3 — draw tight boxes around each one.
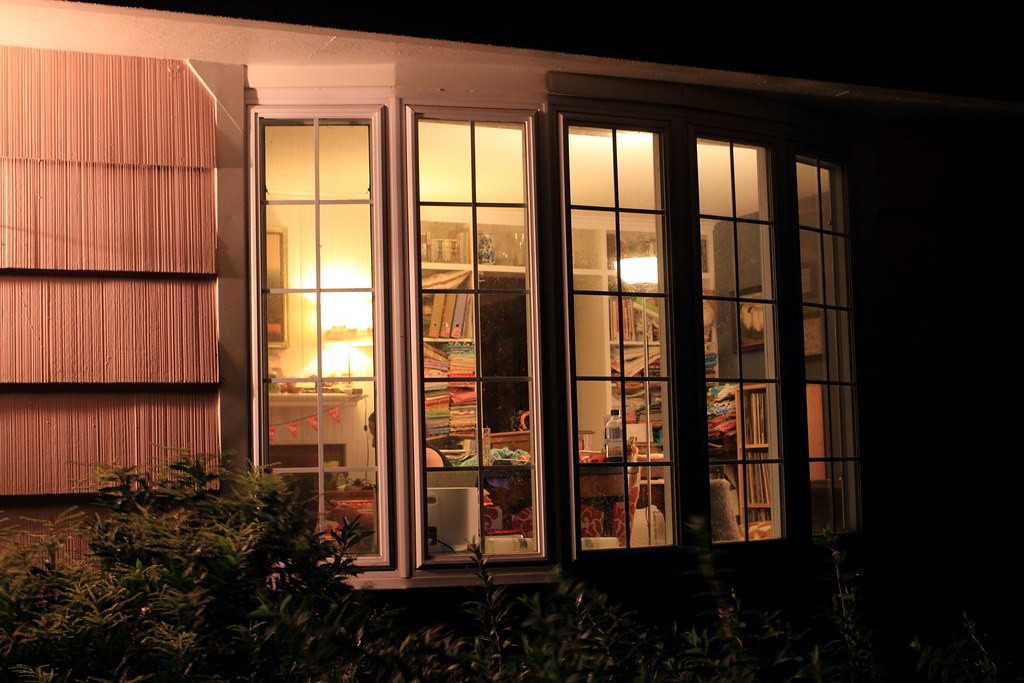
[605,410,623,462]
[479,233,496,264]
[496,233,514,265]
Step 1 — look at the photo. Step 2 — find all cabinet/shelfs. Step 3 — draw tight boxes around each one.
[269,335,372,408]
[418,224,777,553]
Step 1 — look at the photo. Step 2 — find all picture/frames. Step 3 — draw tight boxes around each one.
[801,259,821,299]
[728,283,764,352]
[803,311,823,362]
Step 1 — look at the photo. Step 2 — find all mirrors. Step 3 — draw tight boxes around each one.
[264,228,289,347]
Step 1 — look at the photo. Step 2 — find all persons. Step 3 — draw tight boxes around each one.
[324,412,454,555]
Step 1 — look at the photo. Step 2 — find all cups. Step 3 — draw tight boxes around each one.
[513,233,524,266]
